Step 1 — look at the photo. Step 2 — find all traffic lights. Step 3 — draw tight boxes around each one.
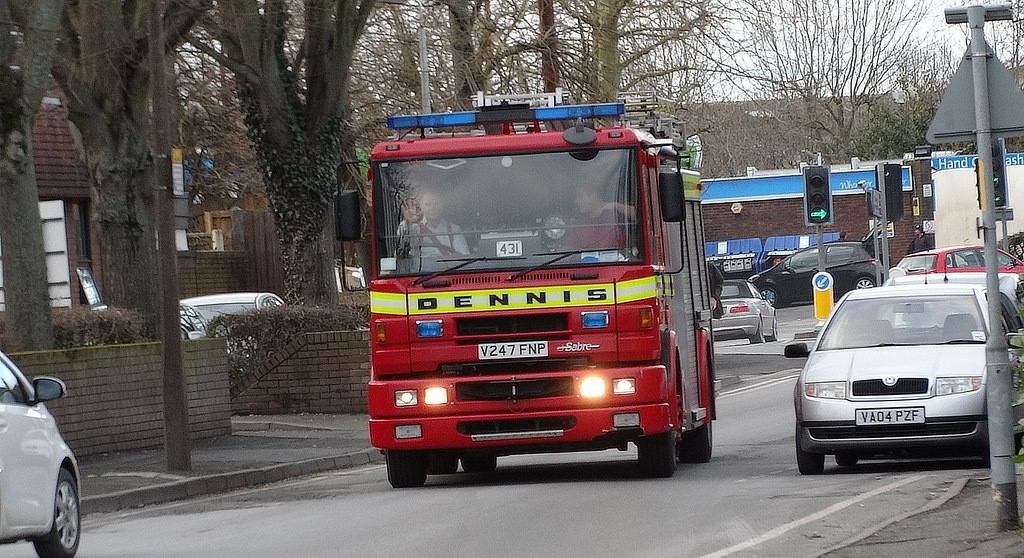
[800,165,832,225]
[973,140,1012,213]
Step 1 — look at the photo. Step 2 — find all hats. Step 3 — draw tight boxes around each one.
[915,224,923,231]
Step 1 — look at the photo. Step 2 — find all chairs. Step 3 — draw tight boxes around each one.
[863,320,896,345]
[963,254,976,267]
[941,314,979,341]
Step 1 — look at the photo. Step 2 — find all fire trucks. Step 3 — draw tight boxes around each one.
[334,88,717,487]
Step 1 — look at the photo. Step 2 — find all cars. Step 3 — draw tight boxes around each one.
[709,275,778,343]
[747,239,886,311]
[0,343,84,558]
[781,282,1008,472]
[892,246,1023,290]
[90,293,293,343]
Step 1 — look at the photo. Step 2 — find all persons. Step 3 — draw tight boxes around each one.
[564,173,637,249]
[907,224,931,255]
[765,257,781,269]
[707,262,725,319]
[837,232,847,241]
[397,191,471,257]
